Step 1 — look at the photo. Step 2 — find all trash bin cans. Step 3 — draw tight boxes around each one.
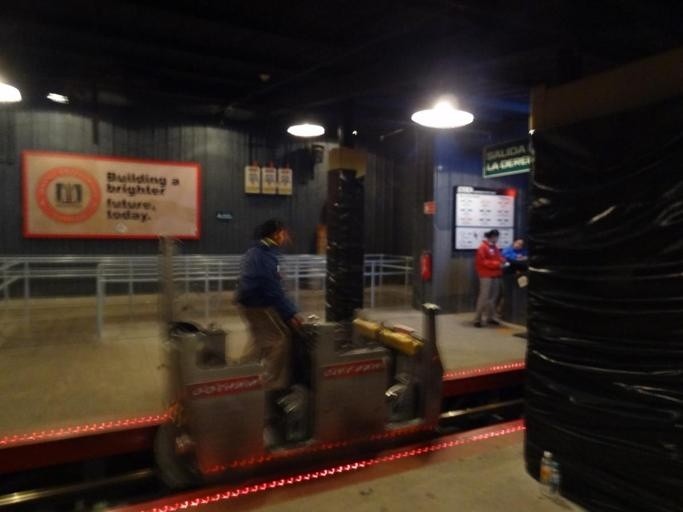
[169,319,227,398]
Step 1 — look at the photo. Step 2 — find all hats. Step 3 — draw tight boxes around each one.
[260,214,288,238]
[484,229,499,238]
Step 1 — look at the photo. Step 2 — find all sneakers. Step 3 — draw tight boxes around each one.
[487,318,500,326]
[474,322,481,327]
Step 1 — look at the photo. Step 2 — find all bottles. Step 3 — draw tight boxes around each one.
[537,451,561,499]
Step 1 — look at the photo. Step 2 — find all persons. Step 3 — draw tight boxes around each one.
[233,216,302,398]
[472,229,507,327]
[496,238,528,316]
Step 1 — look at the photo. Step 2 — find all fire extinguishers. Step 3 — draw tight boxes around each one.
[419,249,432,282]
